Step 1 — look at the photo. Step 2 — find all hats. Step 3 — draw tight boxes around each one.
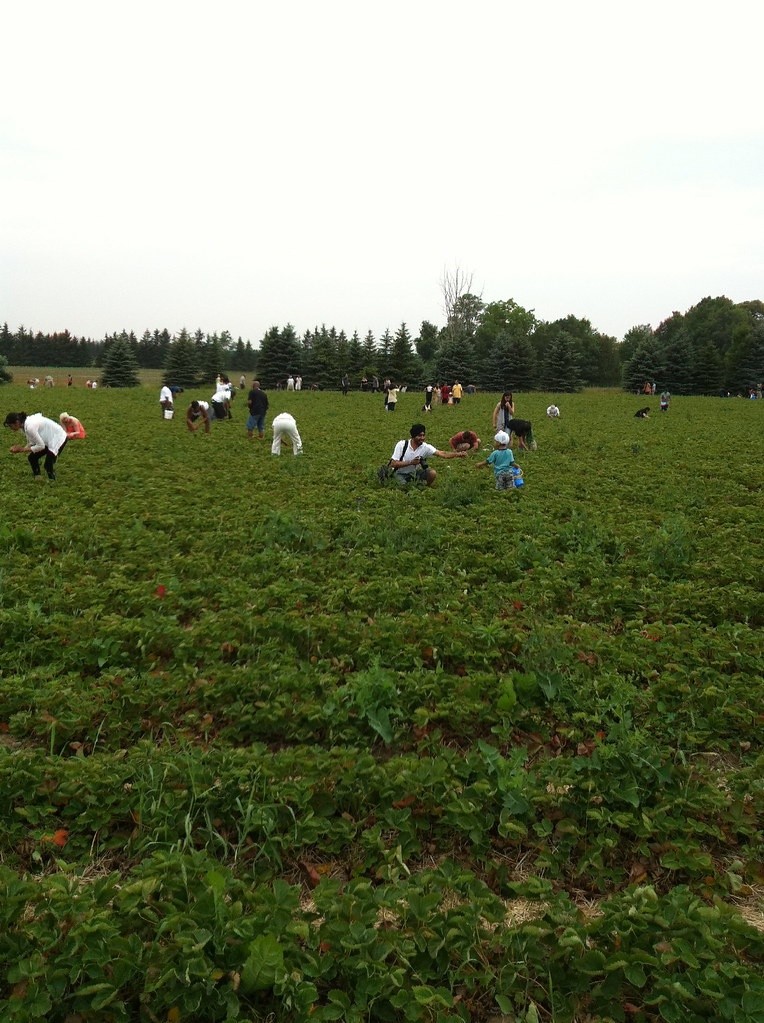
[494,430,510,445]
[410,424,425,438]
[550,405,555,411]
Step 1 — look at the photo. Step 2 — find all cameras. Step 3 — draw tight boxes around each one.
[419,457,428,470]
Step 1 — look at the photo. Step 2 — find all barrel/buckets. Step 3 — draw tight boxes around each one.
[511,466,525,487]
[164,410,174,419]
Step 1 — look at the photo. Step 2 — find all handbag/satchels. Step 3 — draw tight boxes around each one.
[528,440,537,450]
[660,400,670,407]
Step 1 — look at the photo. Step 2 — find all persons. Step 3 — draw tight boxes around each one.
[187,374,247,433]
[361,374,407,410]
[750,388,756,399]
[643,381,656,395]
[159,381,184,420]
[60,413,86,439]
[67,375,72,386]
[271,413,303,455]
[634,407,650,418]
[342,373,350,396]
[4,412,68,481]
[478,433,520,490]
[247,382,268,440]
[492,393,531,450]
[546,405,559,417]
[390,425,468,485]
[84,380,98,388]
[287,375,317,390]
[448,431,481,452]
[661,389,671,412]
[27,375,54,387]
[423,379,476,412]
